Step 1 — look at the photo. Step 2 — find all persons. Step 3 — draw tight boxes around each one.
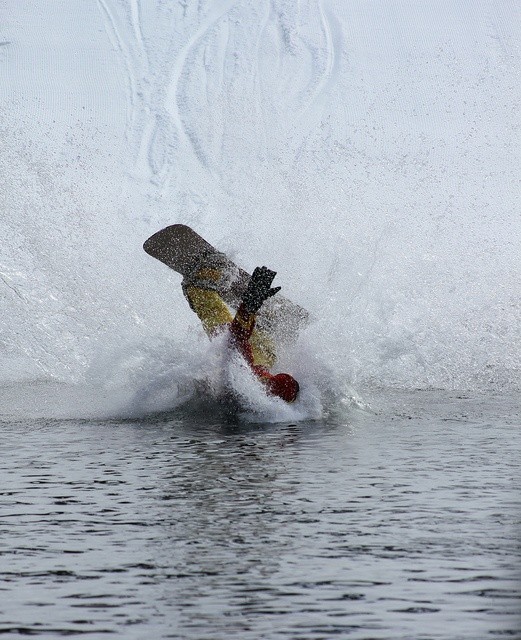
[175,265,300,407]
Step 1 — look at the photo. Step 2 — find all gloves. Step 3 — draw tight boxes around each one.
[242,266,281,314]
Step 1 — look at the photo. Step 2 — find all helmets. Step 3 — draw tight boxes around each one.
[266,374,299,402]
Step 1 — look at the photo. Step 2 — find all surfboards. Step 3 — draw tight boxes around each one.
[142,223,318,349]
